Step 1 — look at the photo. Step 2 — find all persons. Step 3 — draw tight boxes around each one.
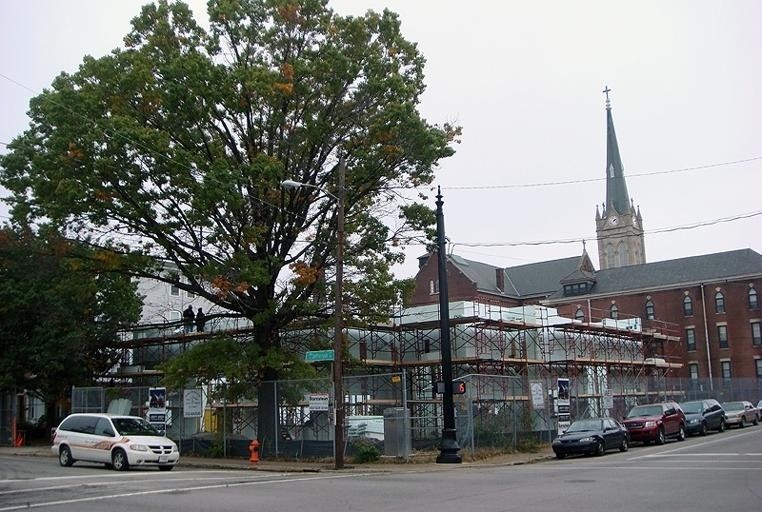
[184,305,195,331]
[196,308,206,333]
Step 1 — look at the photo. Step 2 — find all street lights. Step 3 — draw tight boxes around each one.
[280,159,346,471]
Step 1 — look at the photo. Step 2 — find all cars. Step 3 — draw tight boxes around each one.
[721,400,760,428]
[552,418,629,457]
[677,399,726,434]
[51,413,180,470]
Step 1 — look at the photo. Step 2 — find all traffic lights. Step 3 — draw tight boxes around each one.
[453,381,466,394]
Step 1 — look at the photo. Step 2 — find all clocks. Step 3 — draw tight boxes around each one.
[608,215,619,226]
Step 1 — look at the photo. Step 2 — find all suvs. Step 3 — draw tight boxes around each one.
[620,400,686,445]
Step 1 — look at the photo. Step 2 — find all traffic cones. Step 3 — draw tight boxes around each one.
[16,434,23,447]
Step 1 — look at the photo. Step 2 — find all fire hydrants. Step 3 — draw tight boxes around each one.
[250,440,260,462]
